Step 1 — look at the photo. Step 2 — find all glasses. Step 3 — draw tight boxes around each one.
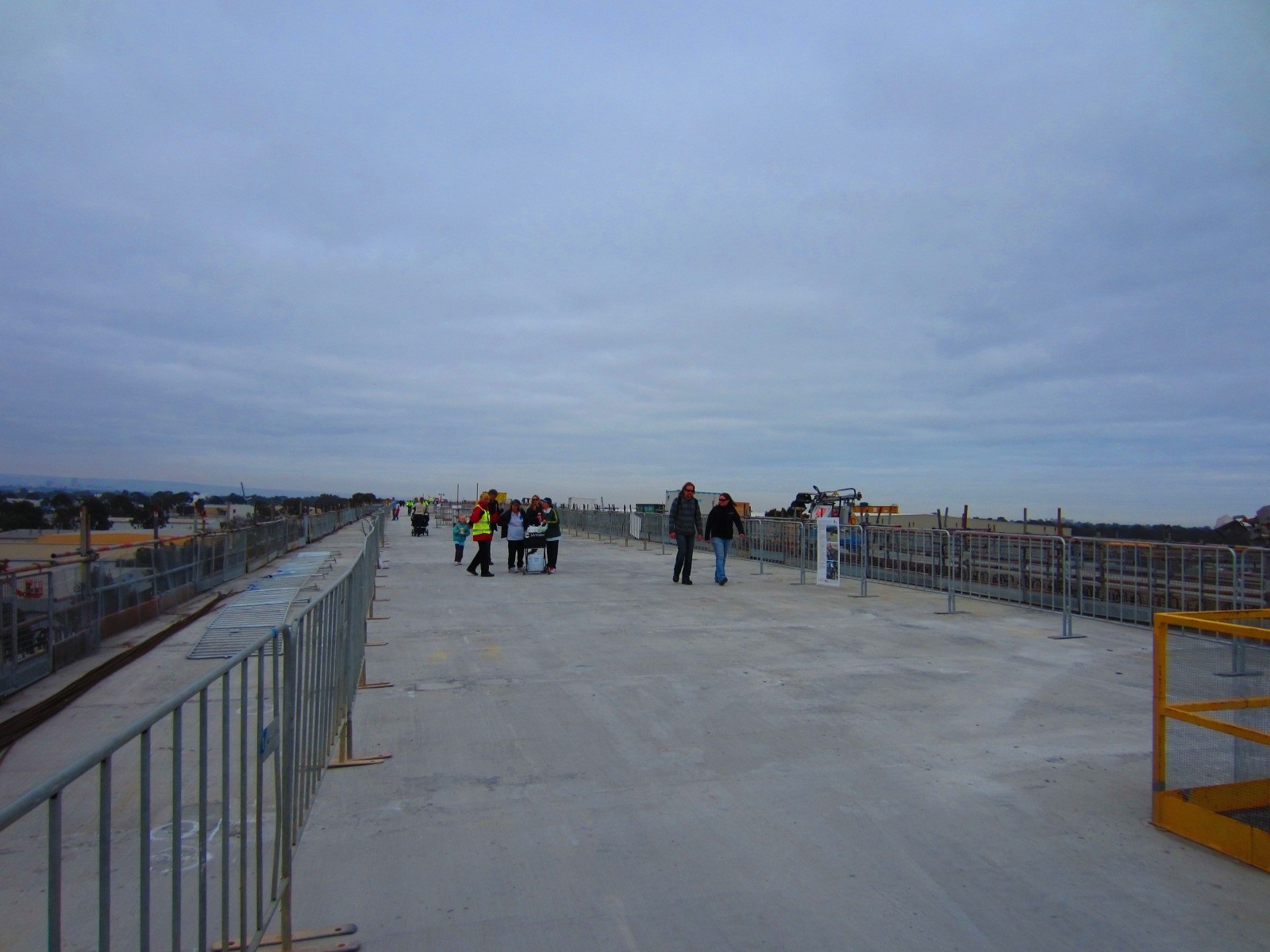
[719,498,728,502]
[533,499,539,501]
[684,489,694,493]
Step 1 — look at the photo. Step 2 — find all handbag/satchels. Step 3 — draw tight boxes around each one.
[525,525,547,572]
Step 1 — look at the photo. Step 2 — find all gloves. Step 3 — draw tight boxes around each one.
[468,521,473,528]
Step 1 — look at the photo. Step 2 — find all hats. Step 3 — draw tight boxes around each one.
[512,499,521,505]
[540,497,552,504]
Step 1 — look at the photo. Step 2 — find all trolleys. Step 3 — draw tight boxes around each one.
[522,532,548,575]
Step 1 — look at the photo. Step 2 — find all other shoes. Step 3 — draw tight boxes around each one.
[488,561,494,565]
[481,571,495,576]
[673,573,679,583]
[517,566,525,572]
[467,568,478,575]
[682,579,692,585]
[508,566,517,573]
[548,567,556,574]
[454,561,462,565]
[715,577,727,585]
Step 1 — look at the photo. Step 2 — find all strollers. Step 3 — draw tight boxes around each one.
[411,513,430,536]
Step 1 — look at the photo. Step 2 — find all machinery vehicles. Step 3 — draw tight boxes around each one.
[809,485,899,527]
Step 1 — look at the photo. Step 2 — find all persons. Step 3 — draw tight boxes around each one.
[390,497,429,520]
[497,499,527,573]
[526,494,546,566]
[541,498,562,574]
[484,488,499,565]
[705,492,746,585]
[668,482,703,585]
[467,492,496,576]
[452,513,470,566]
[410,497,426,536]
